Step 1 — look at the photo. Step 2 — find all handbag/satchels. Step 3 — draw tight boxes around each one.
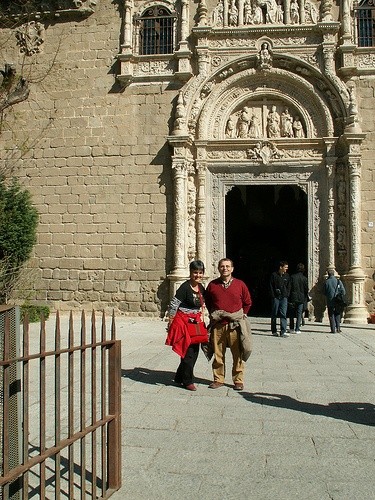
[335,279,348,307]
[187,322,208,344]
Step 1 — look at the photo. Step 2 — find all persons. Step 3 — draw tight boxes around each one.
[200,258,252,390]
[166,261,207,392]
[270,262,308,337]
[325,270,345,334]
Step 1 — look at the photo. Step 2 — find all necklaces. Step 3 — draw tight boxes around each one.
[220,277,232,287]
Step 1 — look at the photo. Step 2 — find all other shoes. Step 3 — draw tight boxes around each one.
[302,321,305,326]
[337,329,342,333]
[290,330,295,333]
[273,333,278,336]
[183,382,196,391]
[330,332,334,333]
[296,331,301,333]
[208,382,225,389]
[280,331,290,337]
[233,383,243,389]
